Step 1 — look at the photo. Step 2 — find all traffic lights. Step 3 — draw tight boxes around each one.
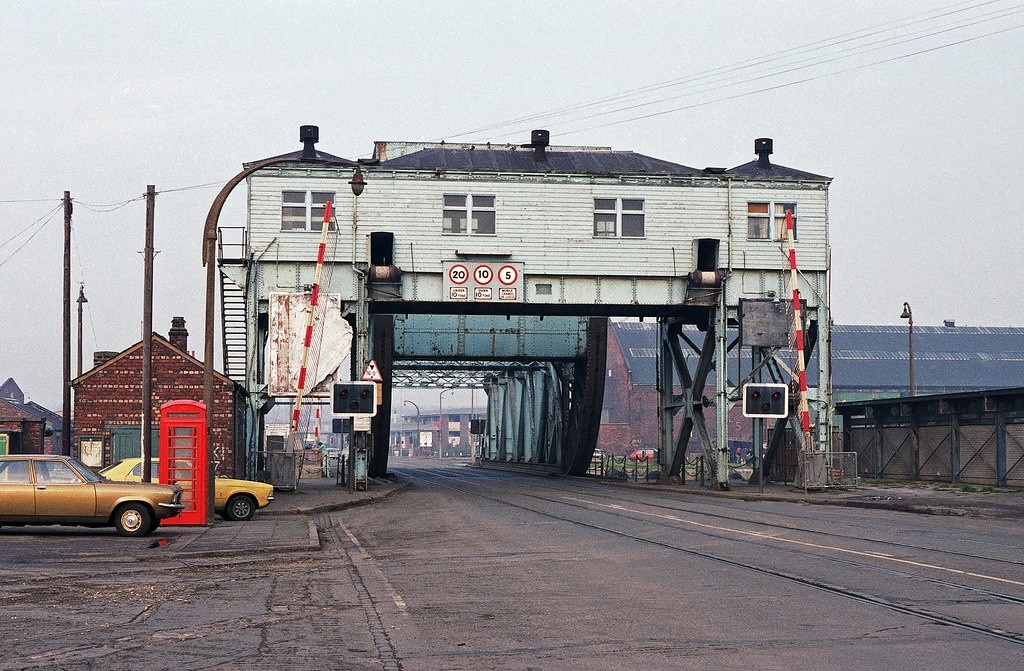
[331,382,376,417]
[743,383,789,418]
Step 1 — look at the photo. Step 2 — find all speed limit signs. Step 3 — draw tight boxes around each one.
[473,265,494,286]
[497,266,518,286]
[450,265,469,285]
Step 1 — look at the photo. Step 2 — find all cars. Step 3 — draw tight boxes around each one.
[629,448,658,463]
[591,449,606,463]
[93,458,275,521]
[0,454,186,538]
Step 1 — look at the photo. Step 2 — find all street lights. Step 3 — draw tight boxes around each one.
[404,400,419,448]
[899,301,915,397]
[439,388,455,458]
